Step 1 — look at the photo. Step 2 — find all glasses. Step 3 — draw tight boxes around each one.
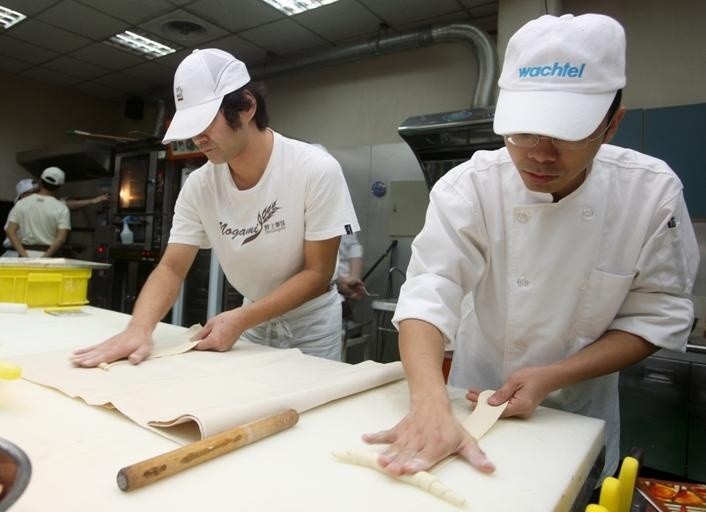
[505,118,613,153]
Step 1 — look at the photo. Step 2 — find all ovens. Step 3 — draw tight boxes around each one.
[86,139,172,328]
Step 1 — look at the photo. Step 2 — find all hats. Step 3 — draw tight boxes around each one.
[161,47,252,146]
[492,12,628,142]
[14,166,66,202]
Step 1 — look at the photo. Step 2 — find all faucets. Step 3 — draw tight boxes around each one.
[388,266,405,279]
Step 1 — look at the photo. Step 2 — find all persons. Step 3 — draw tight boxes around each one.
[363,13,700,497]
[1,167,110,257]
[70,48,363,368]
[335,232,362,286]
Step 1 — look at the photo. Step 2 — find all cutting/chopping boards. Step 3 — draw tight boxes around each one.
[0,301,606,512]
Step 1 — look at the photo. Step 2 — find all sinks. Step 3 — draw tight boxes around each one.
[372,296,396,312]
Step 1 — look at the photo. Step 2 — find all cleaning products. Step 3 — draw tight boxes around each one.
[120,216,134,245]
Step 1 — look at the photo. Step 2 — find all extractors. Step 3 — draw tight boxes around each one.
[16,140,111,183]
[398,105,507,197]
